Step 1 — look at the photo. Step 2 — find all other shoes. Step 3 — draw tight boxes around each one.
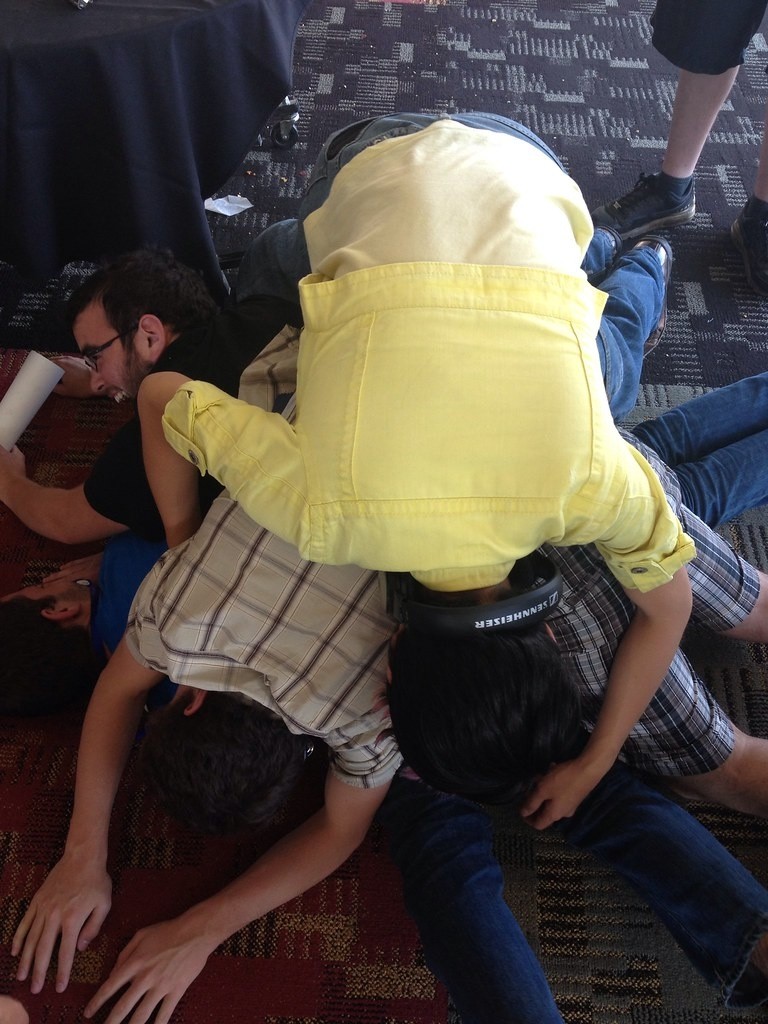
[633,234,674,361]
[596,225,621,258]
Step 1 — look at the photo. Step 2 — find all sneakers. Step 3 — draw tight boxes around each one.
[593,171,696,239]
[731,211,768,295]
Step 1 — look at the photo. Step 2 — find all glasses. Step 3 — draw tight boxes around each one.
[83,327,131,372]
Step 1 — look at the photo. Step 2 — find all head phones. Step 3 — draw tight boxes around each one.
[379,550,563,638]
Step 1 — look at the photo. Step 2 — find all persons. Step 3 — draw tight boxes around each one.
[588,0,768,299]
[0,110,767,1024]
